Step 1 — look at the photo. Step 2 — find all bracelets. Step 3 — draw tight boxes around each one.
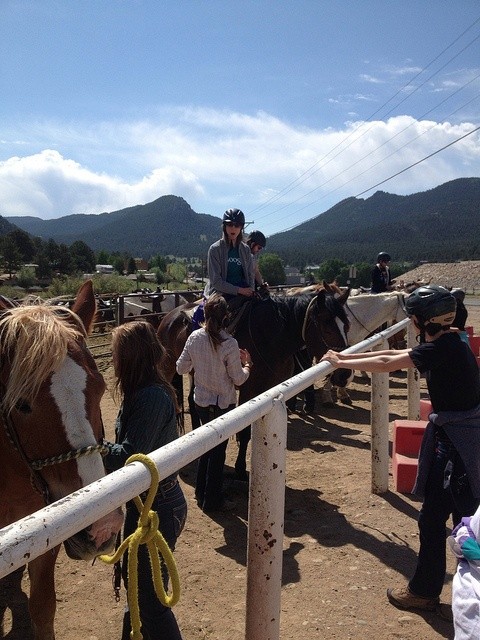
[243,365,251,370]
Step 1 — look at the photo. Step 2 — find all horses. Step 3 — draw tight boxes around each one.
[321,291,411,406]
[0,279,127,640]
[155,279,355,482]
[349,277,434,298]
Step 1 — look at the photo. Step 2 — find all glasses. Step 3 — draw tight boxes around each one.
[226,223,242,228]
[384,262,386,263]
[258,247,262,250]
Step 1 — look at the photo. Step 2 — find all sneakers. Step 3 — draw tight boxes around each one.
[223,496,238,511]
[387,588,440,612]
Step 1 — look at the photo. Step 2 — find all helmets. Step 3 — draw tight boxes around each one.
[249,230,266,247]
[378,252,391,261]
[450,287,465,301]
[222,208,245,224]
[403,284,457,330]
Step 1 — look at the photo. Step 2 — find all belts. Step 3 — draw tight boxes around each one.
[140,477,177,498]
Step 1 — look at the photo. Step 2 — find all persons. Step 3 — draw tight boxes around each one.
[318,285,480,612]
[98,321,188,640]
[450,288,471,348]
[202,209,256,310]
[175,294,254,514]
[243,231,269,289]
[370,251,396,294]
